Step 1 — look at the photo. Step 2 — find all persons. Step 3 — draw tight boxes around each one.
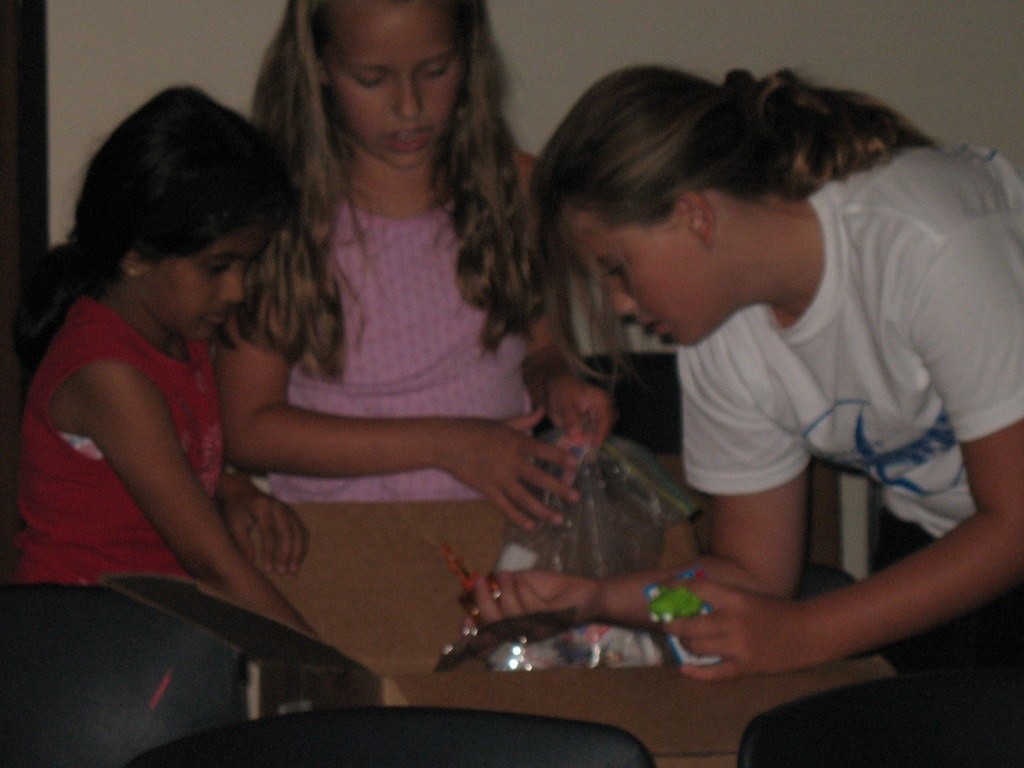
[212,0,622,533]
[11,87,319,643]
[458,61,1024,687]
